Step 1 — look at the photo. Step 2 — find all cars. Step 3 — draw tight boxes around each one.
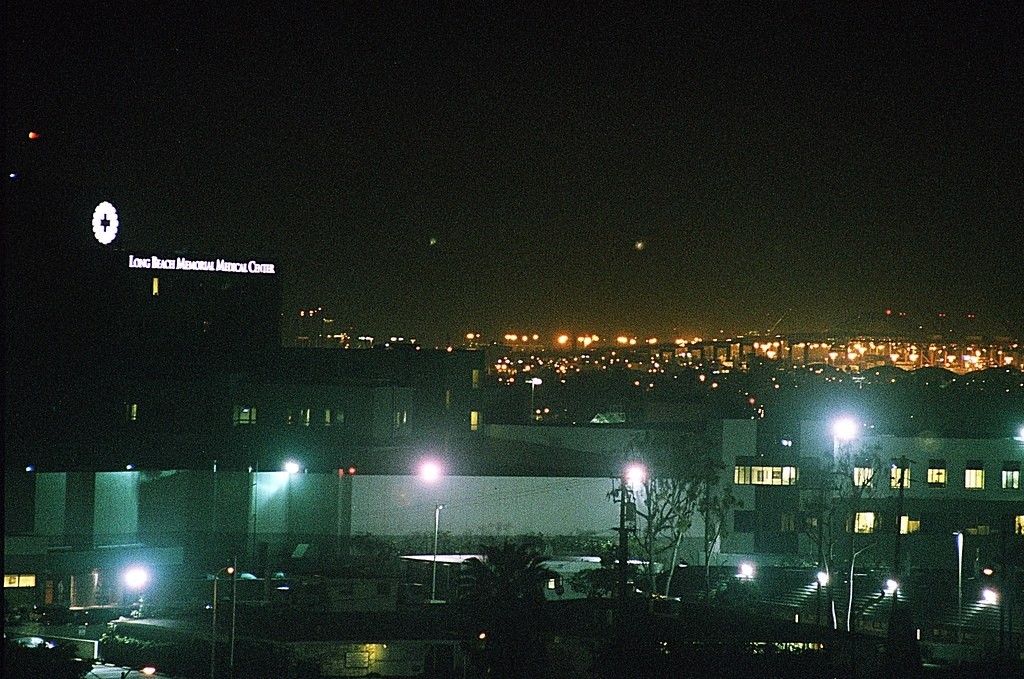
[30,603,72,626]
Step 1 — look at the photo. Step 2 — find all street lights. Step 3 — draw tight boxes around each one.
[211,566,233,676]
[283,460,300,551]
